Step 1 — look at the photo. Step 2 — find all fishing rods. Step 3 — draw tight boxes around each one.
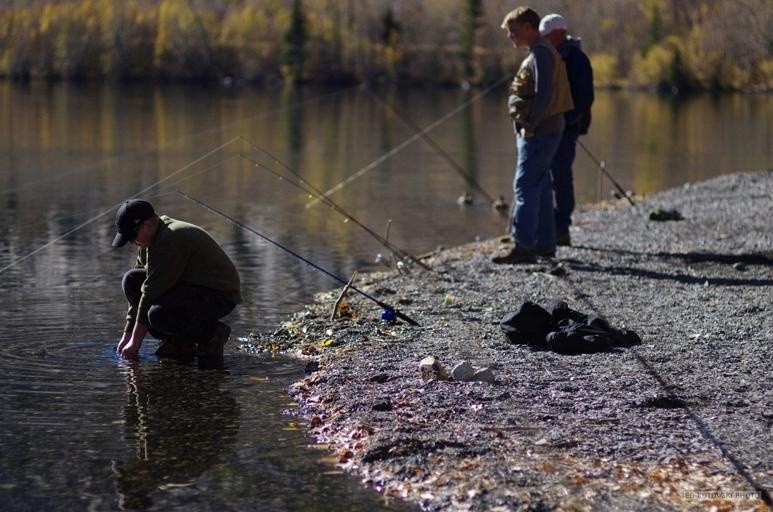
[359,83,510,219]
[240,155,415,279]
[578,141,638,211]
[237,135,433,270]
[175,191,419,325]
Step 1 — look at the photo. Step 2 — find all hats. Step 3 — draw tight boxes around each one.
[111,199,154,247]
[538,13,568,37]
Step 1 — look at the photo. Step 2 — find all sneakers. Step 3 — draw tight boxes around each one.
[492,235,571,263]
[154,321,231,371]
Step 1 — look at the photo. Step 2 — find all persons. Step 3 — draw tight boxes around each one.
[537,13,594,246]
[110,198,243,371]
[489,4,576,263]
